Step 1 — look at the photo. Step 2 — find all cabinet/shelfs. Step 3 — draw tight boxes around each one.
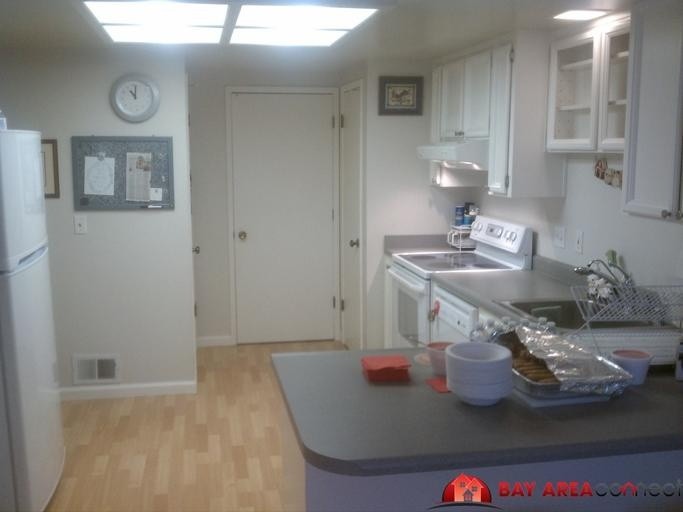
[431,64,441,144]
[431,277,479,343]
[488,43,512,193]
[621,1,682,222]
[545,16,632,152]
[441,50,489,138]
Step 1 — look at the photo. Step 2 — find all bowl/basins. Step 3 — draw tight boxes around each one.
[444,342,513,406]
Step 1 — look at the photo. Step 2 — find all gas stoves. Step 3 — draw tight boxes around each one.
[391,250,522,280]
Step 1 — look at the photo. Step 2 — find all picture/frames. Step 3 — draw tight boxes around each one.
[71,136,175,212]
[41,139,60,199]
[378,76,423,116]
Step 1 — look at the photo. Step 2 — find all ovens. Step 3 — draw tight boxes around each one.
[385,262,430,349]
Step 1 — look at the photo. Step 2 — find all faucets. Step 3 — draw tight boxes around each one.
[573,259,637,308]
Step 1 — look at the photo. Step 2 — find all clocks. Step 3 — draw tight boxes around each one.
[109,75,157,123]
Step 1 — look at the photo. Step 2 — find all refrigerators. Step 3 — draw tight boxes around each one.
[0,129,67,512]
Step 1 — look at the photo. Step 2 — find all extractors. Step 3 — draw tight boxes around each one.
[415,142,488,172]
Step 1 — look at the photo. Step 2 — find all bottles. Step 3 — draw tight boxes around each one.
[674,342,683,380]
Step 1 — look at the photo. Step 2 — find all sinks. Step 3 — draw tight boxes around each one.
[491,297,679,333]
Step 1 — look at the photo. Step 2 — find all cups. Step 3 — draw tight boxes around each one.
[610,348,654,385]
[425,341,454,376]
